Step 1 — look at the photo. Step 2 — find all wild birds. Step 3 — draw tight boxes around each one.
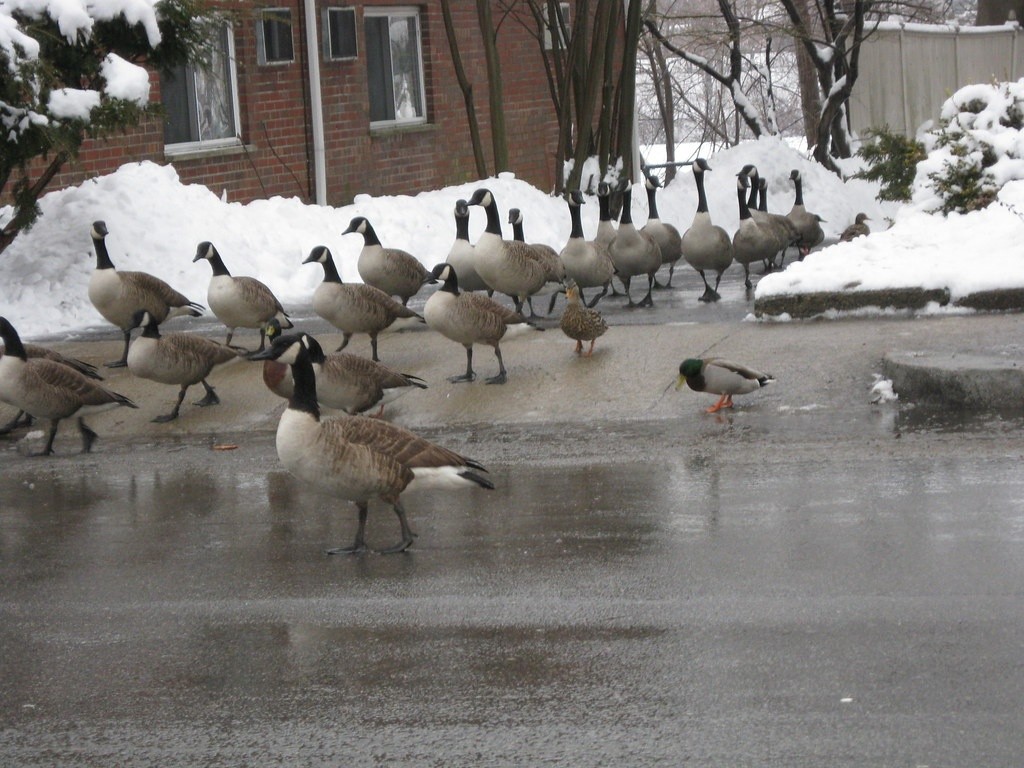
[839,212,873,243]
[192,241,295,357]
[341,216,440,307]
[302,245,427,362]
[122,309,248,424]
[445,158,829,358]
[422,263,527,386]
[88,220,206,369]
[0,316,141,458]
[248,319,496,556]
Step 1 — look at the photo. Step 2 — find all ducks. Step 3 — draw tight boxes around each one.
[675,358,780,414]
